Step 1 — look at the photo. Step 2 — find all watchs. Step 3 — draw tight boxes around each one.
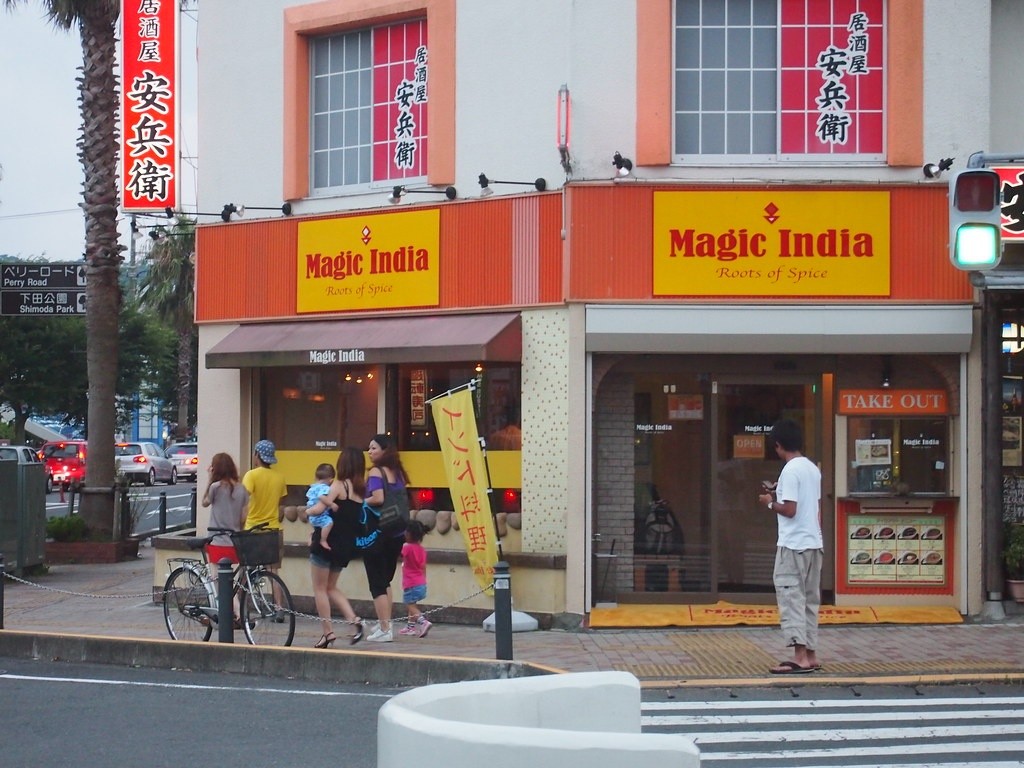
[768,501,775,510]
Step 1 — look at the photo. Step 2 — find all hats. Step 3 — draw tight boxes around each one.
[255,440,277,463]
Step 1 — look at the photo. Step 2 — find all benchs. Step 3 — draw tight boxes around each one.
[632,554,681,592]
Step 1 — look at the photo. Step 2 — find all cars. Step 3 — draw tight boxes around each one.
[0,445,54,494]
[36,441,88,493]
[115,442,179,486]
[163,441,198,482]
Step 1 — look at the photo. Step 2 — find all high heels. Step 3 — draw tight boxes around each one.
[315,632,337,649]
[350,622,364,646]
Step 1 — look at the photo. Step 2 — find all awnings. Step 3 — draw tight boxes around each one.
[205,313,518,370]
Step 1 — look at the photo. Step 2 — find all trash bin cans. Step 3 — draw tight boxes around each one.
[0,459,45,580]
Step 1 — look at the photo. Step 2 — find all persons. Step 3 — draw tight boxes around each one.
[306,449,367,649]
[759,420,825,672]
[368,434,410,641]
[400,520,432,637]
[199,454,249,621]
[306,463,338,550]
[242,440,287,622]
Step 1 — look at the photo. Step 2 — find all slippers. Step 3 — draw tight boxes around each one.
[811,664,821,670]
[769,662,813,674]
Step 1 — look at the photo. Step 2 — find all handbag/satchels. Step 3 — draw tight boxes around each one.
[356,496,383,552]
[367,465,404,529]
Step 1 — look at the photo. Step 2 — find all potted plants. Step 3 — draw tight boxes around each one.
[1001,533,1024,603]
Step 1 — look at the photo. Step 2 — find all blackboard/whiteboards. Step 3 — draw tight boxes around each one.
[1001,475,1024,527]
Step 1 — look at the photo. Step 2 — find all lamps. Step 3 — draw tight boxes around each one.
[478,174,546,198]
[923,157,956,179]
[387,185,456,203]
[130,221,196,239]
[164,205,230,225]
[345,372,373,383]
[612,151,633,178]
[149,231,196,244]
[225,204,291,218]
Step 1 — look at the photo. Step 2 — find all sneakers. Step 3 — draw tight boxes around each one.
[371,622,392,633]
[418,620,433,638]
[367,628,393,642]
[400,626,417,636]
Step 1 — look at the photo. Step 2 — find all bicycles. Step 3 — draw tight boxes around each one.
[163,522,298,648]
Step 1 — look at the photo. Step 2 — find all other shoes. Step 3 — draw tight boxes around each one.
[272,610,285,624]
[237,616,256,630]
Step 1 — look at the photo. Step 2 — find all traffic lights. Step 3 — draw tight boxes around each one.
[950,168,1006,275]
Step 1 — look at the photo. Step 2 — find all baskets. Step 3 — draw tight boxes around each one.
[230,530,284,565]
